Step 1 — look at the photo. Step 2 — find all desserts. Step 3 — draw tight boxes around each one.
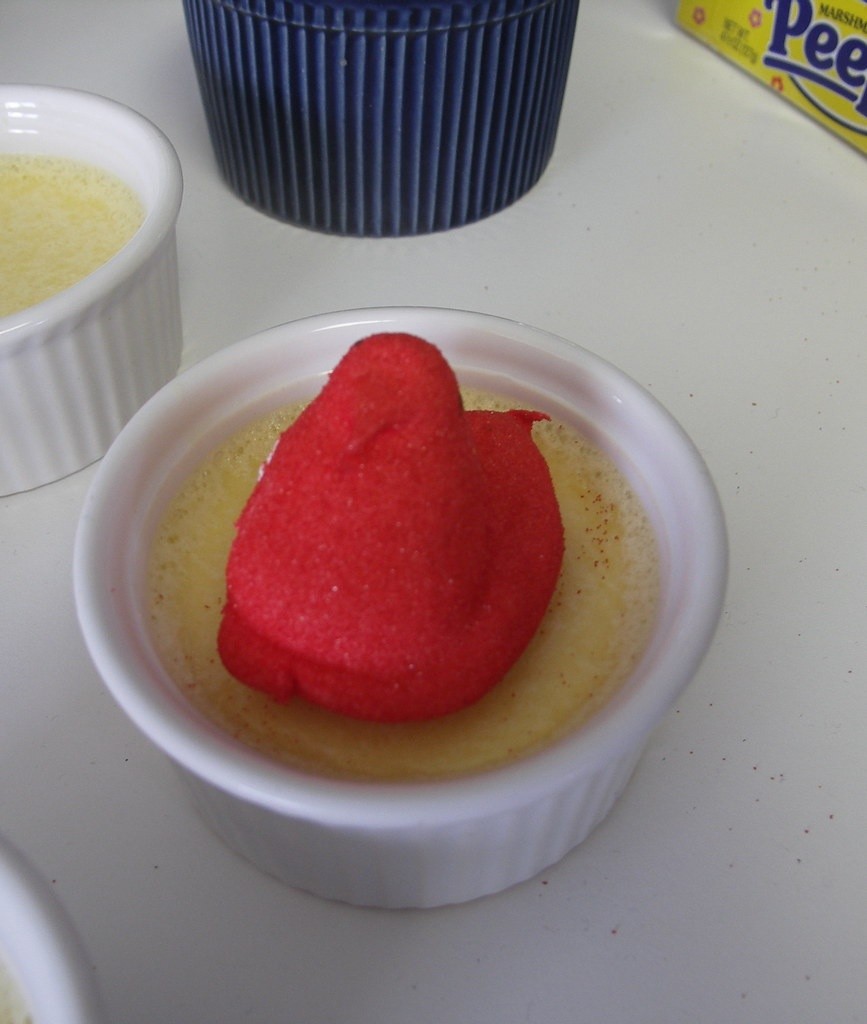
[154,331,660,782]
[0,150,144,320]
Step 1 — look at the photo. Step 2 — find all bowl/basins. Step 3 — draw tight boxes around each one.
[0,834,106,1024]
[184,0,579,239]
[0,85,184,497]
[73,301,731,913]
